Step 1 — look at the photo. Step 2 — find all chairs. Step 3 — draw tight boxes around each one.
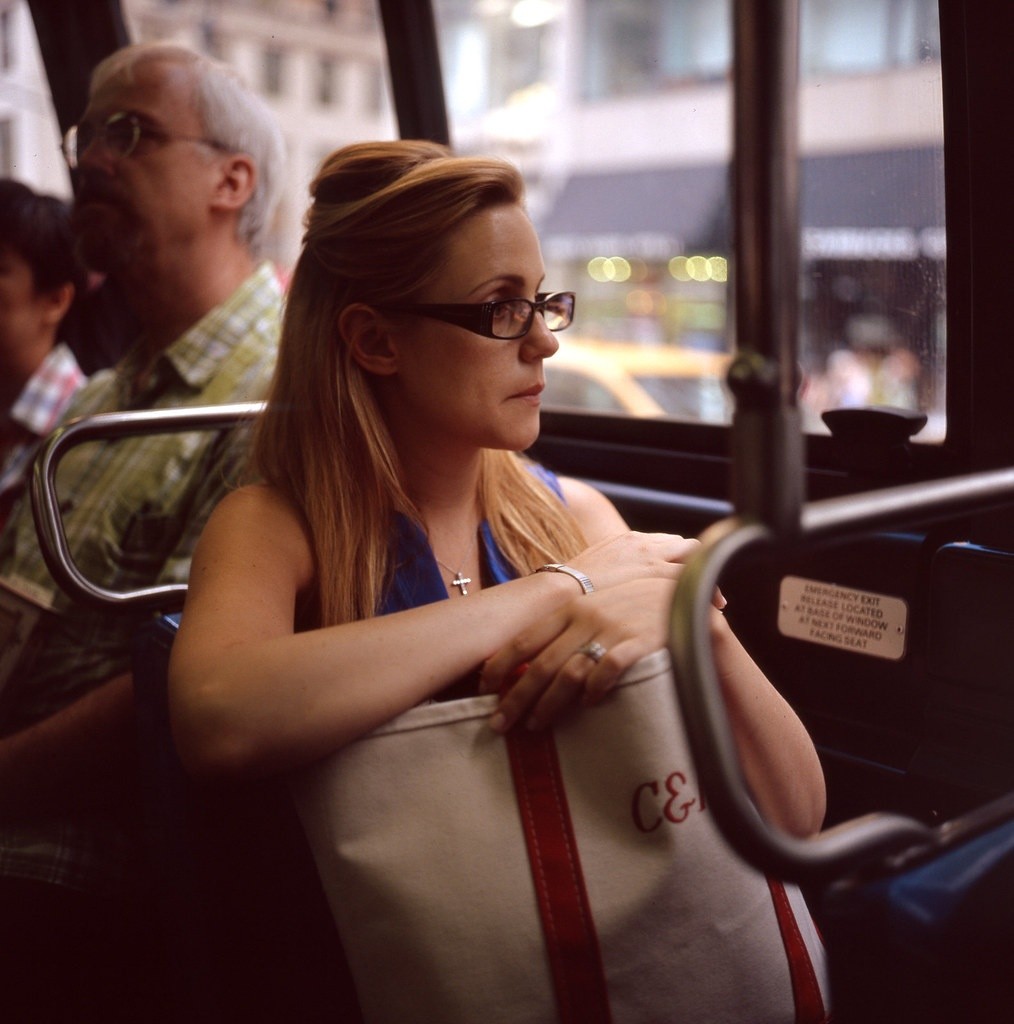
[33,409,1014,1024]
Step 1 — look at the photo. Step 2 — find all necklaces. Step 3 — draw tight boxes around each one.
[437,544,471,596]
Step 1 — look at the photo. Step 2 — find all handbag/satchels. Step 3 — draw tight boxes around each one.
[285,645,830,1024]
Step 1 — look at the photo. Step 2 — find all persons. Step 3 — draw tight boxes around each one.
[0,179,91,498]
[171,138,826,841]
[827,315,918,409]
[0,41,290,1024]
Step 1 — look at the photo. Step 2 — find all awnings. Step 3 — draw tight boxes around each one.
[540,147,947,261]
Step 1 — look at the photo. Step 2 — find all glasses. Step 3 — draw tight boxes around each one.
[392,290,576,341]
[59,111,229,172]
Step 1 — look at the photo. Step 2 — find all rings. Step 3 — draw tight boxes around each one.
[580,641,607,661]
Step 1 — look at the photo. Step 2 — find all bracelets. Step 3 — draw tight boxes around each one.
[535,563,594,594]
